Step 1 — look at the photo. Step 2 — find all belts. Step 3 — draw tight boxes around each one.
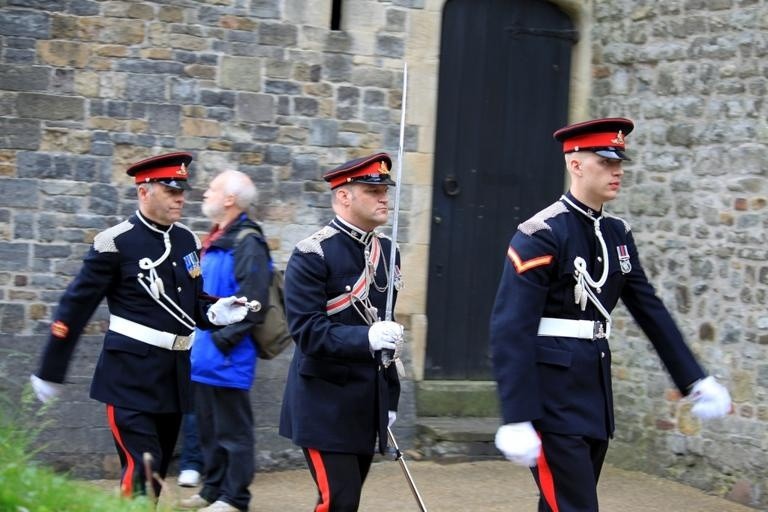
[108,314,195,351]
[537,318,611,342]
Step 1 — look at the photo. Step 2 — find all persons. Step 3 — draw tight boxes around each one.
[489,116,731,511]
[277,152,405,512]
[31,152,249,512]
[177,410,206,488]
[177,170,270,512]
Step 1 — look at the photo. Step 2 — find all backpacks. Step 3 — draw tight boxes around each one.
[233,228,292,359]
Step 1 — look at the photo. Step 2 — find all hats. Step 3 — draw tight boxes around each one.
[322,152,396,190]
[126,152,192,191]
[553,117,633,160]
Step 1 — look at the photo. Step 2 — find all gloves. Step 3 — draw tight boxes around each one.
[687,375,732,420]
[494,420,542,468]
[30,373,64,403]
[206,296,248,326]
[368,320,404,351]
[387,410,397,427]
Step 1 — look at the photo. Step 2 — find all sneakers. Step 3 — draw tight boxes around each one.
[177,469,206,487]
[170,494,240,512]
